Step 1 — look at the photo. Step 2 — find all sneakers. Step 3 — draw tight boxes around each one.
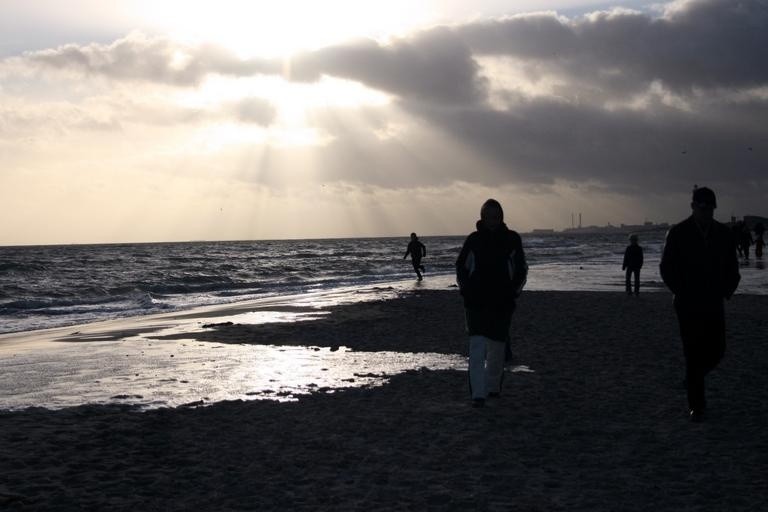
[471,391,500,408]
[689,411,707,422]
[705,353,727,376]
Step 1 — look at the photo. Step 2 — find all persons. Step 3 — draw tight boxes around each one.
[454,197,528,407]
[402,232,427,282]
[658,186,743,425]
[621,233,644,297]
[729,219,766,261]
